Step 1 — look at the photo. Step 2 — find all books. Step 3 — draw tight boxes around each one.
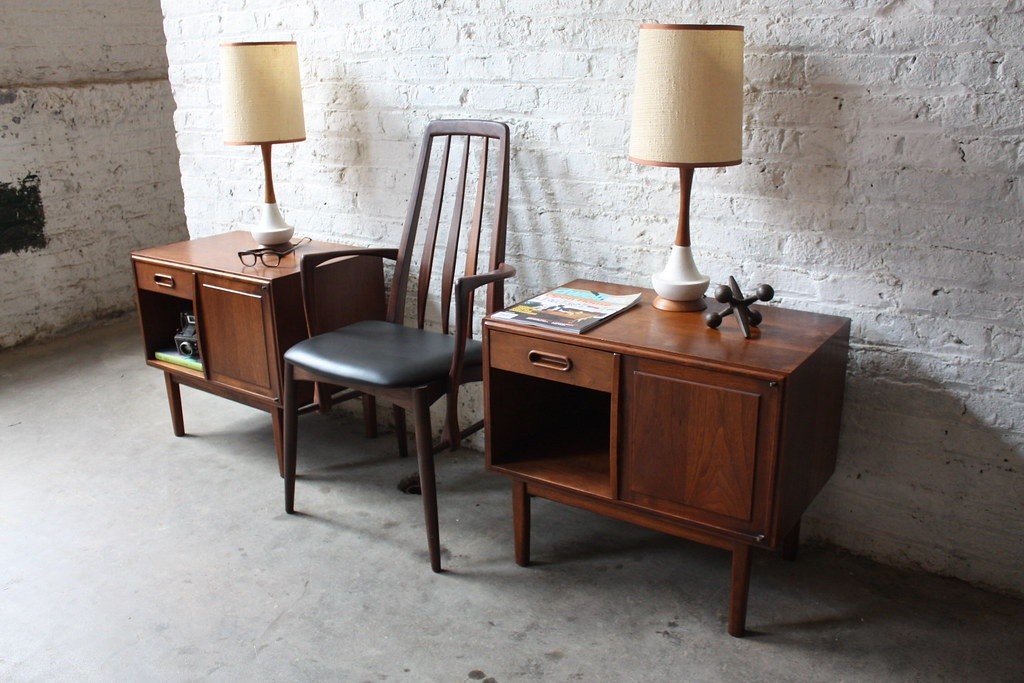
[491,286,642,334]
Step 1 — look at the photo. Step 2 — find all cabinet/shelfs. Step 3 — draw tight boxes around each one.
[482,277,852,636]
[129,231,379,478]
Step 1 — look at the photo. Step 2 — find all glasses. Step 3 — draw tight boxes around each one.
[238,237,312,267]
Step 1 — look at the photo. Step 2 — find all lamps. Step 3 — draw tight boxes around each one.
[219,41,307,247]
[627,23,745,314]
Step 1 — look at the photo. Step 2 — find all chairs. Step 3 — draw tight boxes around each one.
[284,119,516,572]
